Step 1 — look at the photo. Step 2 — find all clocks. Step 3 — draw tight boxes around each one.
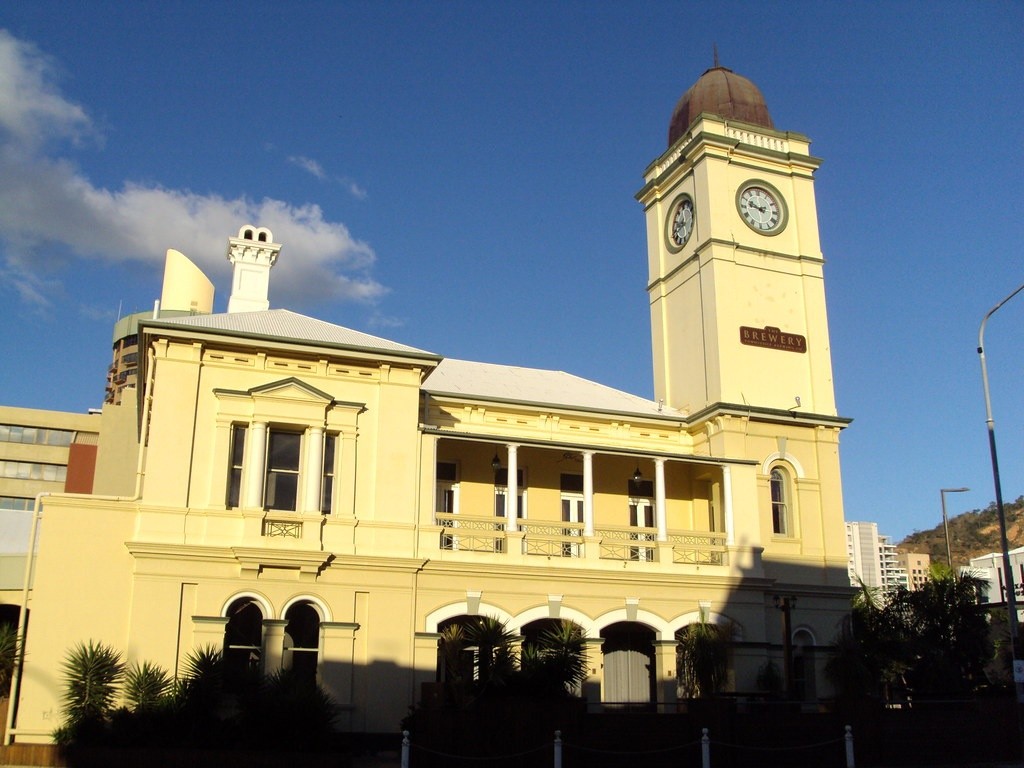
[741,188,779,230]
[673,201,694,245]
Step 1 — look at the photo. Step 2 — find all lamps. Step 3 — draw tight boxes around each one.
[490,446,501,469]
[624,598,639,606]
[466,589,482,597]
[548,594,564,602]
[634,466,644,480]
